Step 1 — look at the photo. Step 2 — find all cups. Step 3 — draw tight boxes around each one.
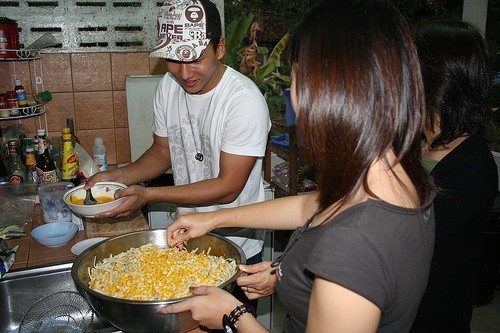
[38,182,74,224]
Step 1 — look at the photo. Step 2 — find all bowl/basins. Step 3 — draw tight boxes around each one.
[63,182,127,218]
[71,229,246,333]
[32,222,78,248]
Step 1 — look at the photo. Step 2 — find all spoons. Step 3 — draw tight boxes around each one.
[84,178,97,205]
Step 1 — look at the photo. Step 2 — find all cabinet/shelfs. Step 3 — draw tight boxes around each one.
[264,119,299,247]
[0,49,48,137]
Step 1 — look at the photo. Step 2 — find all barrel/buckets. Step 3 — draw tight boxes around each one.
[0,17,21,58]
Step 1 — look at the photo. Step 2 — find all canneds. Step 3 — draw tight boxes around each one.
[5,90,20,117]
[0,18,20,58]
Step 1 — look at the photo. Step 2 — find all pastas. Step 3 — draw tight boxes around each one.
[88,242,239,301]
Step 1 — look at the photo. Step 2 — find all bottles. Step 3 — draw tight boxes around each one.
[28,91,52,106]
[7,129,60,196]
[93,138,107,172]
[0,91,20,118]
[58,118,81,188]
[15,80,29,115]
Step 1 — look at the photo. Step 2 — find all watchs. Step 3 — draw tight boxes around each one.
[222,305,252,333]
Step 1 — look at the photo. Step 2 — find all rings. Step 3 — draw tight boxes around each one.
[247,287,249,292]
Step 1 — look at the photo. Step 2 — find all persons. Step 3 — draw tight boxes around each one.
[84,0,273,317]
[236,23,500,333]
[156,0,436,333]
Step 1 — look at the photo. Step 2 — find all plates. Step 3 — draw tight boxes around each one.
[71,238,109,255]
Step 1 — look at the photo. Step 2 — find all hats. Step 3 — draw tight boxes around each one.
[148,0,222,62]
